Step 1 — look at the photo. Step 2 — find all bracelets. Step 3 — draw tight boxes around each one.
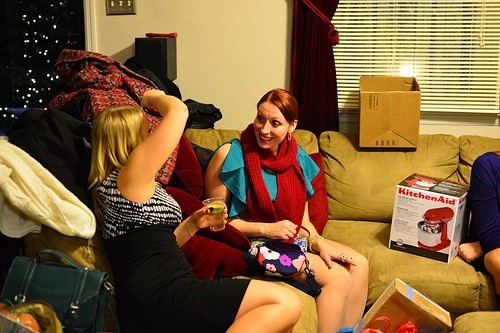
[308,236,325,253]
[187,217,193,238]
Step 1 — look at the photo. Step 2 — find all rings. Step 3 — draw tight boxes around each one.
[342,254,349,262]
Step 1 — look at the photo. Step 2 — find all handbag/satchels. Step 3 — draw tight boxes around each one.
[254,238,307,280]
[0,248,114,333]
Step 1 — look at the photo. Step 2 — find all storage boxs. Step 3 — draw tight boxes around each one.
[388,173,472,263]
[355,278,452,333]
[358,76,421,152]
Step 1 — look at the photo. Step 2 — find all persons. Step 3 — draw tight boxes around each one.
[204,89,369,333]
[89,89,301,333]
[459,152,500,297]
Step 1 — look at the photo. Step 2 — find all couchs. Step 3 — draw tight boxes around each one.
[24,129,500,333]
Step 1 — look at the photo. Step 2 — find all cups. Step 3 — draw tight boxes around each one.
[202,197,226,231]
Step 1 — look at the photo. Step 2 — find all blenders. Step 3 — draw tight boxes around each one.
[417,207,455,252]
